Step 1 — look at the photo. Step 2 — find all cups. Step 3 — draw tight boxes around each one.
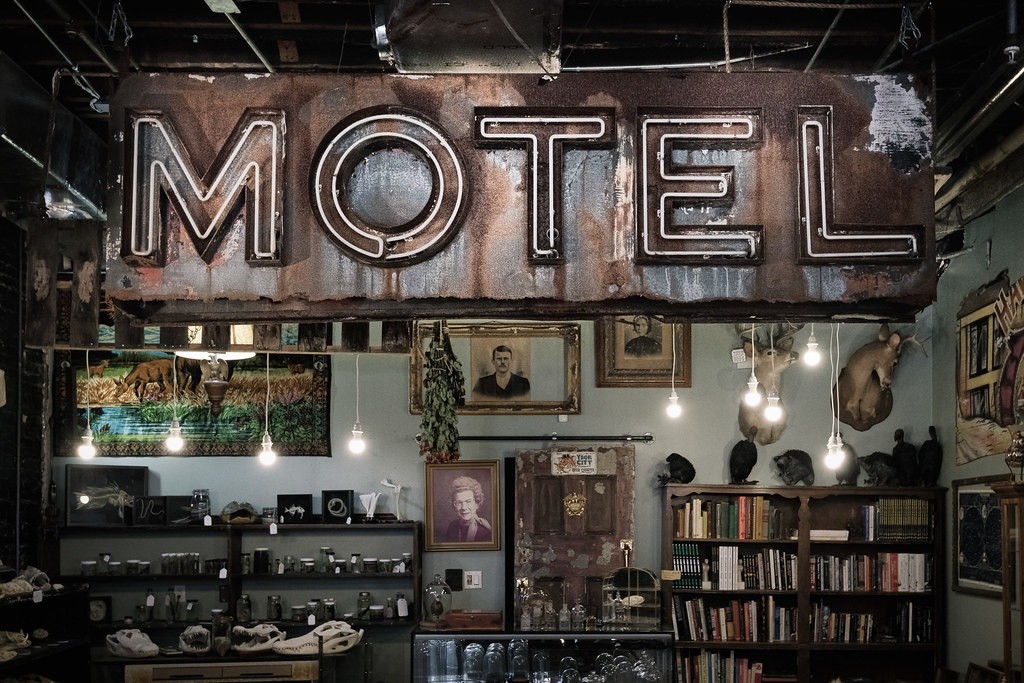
[263,508,276,527]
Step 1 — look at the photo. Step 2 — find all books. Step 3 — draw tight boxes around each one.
[792,529,849,541]
[862,496,934,541]
[685,649,762,683]
[809,552,932,592]
[678,496,783,539]
[813,598,931,643]
[672,544,798,590]
[672,596,798,641]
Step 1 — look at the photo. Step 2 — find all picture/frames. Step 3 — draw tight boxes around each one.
[65,465,146,523]
[954,474,1014,599]
[597,316,691,387]
[424,459,501,552]
[409,324,580,415]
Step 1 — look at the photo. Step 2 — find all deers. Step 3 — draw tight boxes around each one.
[736,319,805,445]
[830,320,932,431]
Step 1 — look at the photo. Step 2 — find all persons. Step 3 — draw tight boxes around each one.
[473,345,529,398]
[625,315,662,356]
[445,476,492,542]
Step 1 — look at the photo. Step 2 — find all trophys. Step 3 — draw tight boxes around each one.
[739,559,745,589]
[702,558,712,590]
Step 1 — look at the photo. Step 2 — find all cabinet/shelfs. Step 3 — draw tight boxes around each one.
[47,525,416,627]
[663,484,949,682]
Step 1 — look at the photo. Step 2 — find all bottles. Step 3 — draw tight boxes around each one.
[242,552,251,575]
[161,552,200,577]
[292,598,335,625]
[267,596,282,623]
[559,601,602,632]
[379,559,391,573]
[205,558,228,578]
[236,594,251,624]
[358,591,409,624]
[81,552,149,577]
[351,553,361,574]
[284,556,294,576]
[363,558,377,574]
[124,586,199,626]
[318,547,347,574]
[521,601,556,632]
[301,558,315,576]
[392,558,402,573]
[275,558,280,575]
[253,548,269,576]
[403,553,412,574]
[190,489,211,525]
[604,592,627,631]
[211,616,230,649]
